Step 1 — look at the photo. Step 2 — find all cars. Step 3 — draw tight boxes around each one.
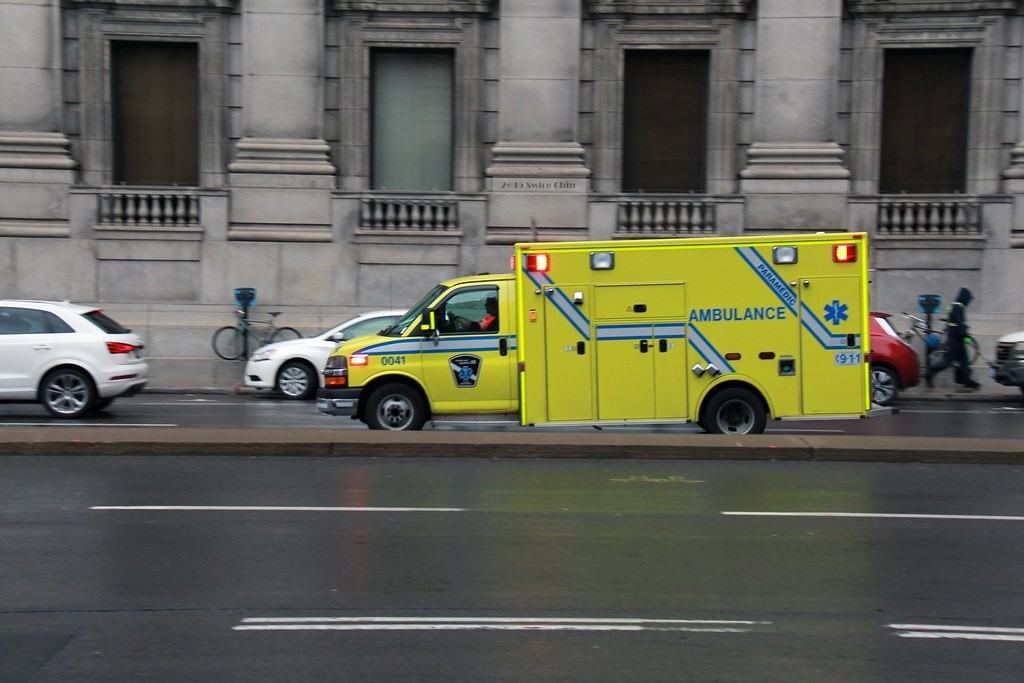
[0,296,150,421]
[870,311,922,407]
[243,308,465,401]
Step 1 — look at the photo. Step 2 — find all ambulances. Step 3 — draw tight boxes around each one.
[318,228,873,437]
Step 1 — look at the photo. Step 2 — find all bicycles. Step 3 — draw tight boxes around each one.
[897,310,982,368]
[210,309,304,361]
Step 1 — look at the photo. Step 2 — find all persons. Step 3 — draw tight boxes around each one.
[451,292,498,332]
[921,287,981,389]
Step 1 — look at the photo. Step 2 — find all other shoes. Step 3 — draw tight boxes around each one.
[924,374,934,387]
[964,380,979,388]
